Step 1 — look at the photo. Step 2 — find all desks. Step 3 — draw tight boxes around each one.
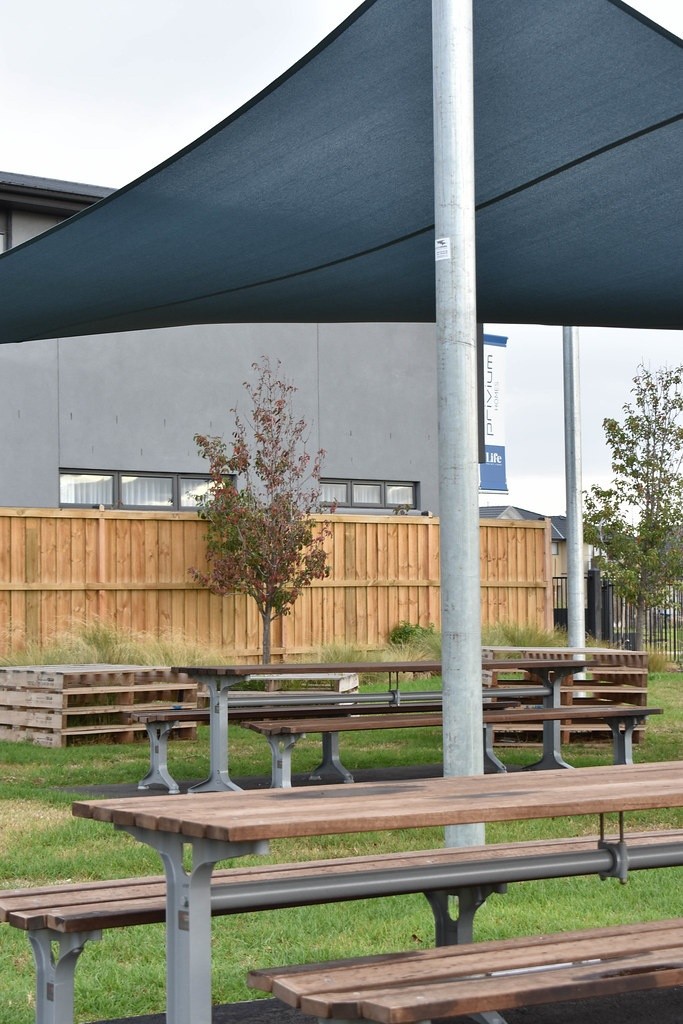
[70,759,683,1024]
[170,659,602,791]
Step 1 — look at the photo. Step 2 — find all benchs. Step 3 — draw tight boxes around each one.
[118,701,524,791]
[0,829,683,944]
[240,701,664,786]
[244,916,683,1024]
